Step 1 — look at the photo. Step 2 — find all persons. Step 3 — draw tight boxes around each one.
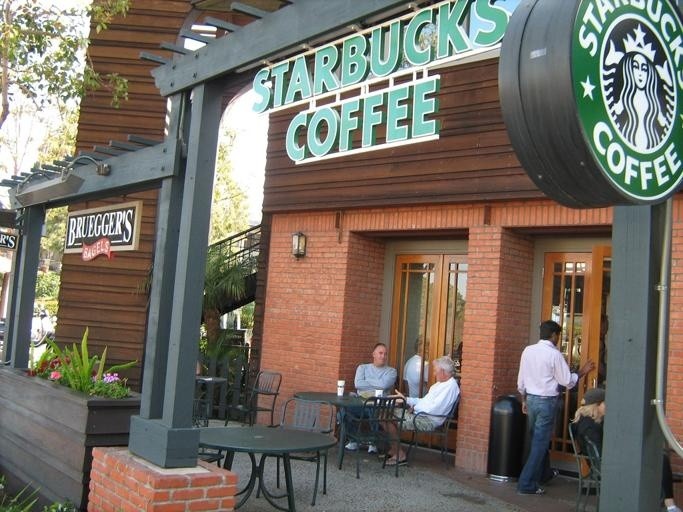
[571,386,682,511]
[375,355,460,469]
[511,318,596,497]
[343,342,399,456]
[401,335,429,400]
[32,310,56,347]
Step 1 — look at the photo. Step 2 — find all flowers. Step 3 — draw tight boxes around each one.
[23,322,140,400]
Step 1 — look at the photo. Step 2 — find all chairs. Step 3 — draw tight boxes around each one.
[193,378,224,468]
[398,378,408,398]
[337,397,406,479]
[223,371,282,426]
[256,398,333,507]
[402,394,460,465]
[567,422,601,511]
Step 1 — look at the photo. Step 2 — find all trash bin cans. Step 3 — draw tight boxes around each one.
[488,396,527,483]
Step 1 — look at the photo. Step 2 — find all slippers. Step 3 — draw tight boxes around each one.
[378,453,392,459]
[385,458,407,464]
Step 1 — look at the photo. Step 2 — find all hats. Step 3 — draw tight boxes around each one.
[580,389,605,406]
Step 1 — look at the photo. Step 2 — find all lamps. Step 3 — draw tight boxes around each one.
[59,155,111,182]
[15,171,51,194]
[290,231,306,259]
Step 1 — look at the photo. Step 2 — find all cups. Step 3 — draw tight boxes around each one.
[374,390,383,404]
[336,380,345,398]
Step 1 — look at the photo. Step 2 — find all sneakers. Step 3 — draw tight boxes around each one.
[345,442,358,450]
[517,488,545,496]
[368,444,378,453]
[536,469,559,487]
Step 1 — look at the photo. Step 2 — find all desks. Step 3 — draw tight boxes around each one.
[199,426,338,512]
[292,391,406,470]
